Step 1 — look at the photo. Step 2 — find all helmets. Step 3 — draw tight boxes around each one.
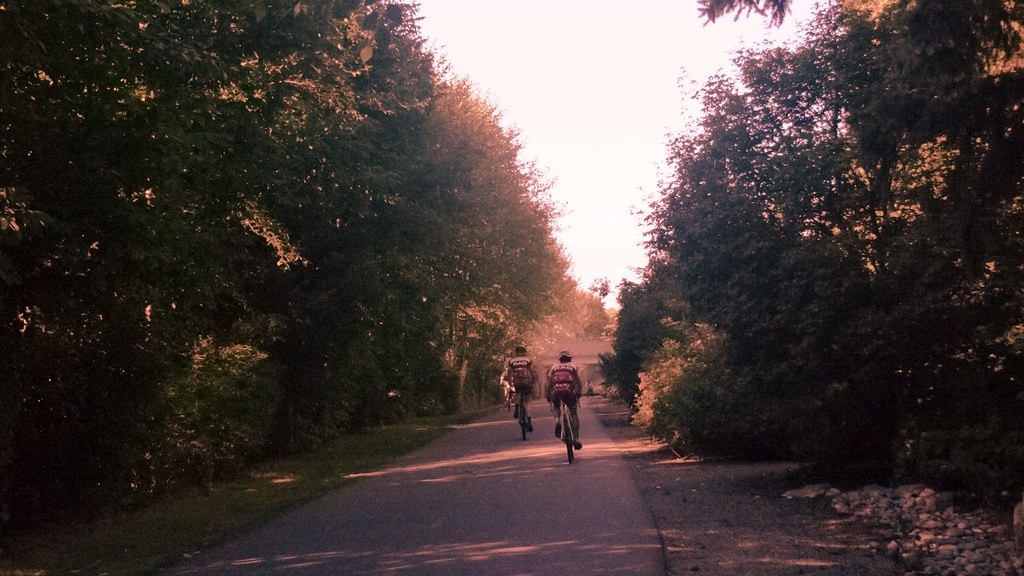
[557,348,572,360]
[516,345,527,351]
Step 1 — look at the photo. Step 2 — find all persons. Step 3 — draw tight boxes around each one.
[508,344,542,431]
[585,380,593,394]
[499,359,517,402]
[545,350,594,450]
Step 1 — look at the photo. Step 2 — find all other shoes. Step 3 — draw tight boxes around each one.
[527,418,534,433]
[574,439,582,449]
[513,402,517,417]
[554,422,562,439]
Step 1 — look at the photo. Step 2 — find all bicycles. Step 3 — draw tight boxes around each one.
[504,373,535,440]
[544,383,582,463]
